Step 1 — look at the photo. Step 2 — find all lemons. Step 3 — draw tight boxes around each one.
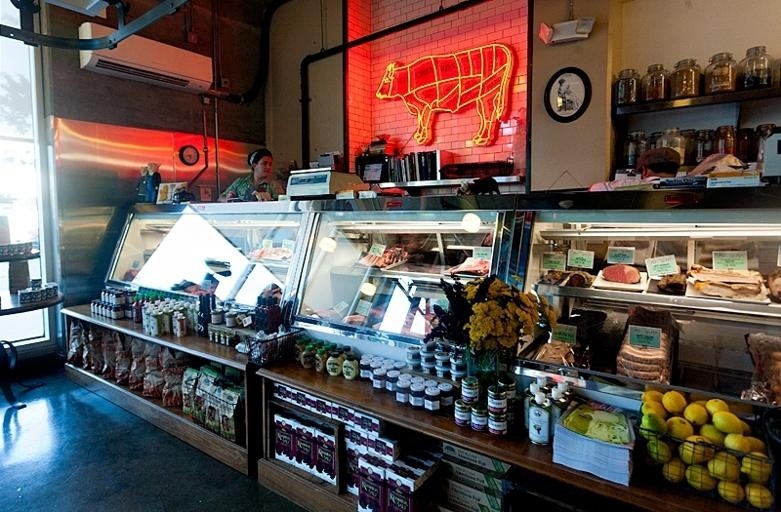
[637,388,774,508]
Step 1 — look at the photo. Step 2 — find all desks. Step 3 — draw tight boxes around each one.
[0,291,64,411]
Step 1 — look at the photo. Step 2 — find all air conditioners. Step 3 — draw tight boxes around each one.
[77,19,213,98]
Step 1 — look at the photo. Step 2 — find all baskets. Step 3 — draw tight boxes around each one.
[632,414,778,511]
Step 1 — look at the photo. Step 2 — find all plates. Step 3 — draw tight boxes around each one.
[685,269,772,307]
[592,267,650,292]
[556,270,597,291]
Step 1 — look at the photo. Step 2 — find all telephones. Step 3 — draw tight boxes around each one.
[173,192,195,203]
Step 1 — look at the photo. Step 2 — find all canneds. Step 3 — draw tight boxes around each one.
[624,124,776,169]
[360,346,517,437]
[91,285,138,320]
[207,307,255,347]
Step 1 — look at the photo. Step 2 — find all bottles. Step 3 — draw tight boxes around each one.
[286,334,360,383]
[614,45,779,171]
[132,292,283,346]
[523,373,574,448]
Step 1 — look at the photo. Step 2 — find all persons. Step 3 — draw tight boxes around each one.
[141,163,161,203]
[216,148,284,202]
[556,78,577,111]
[134,166,147,202]
[586,147,680,193]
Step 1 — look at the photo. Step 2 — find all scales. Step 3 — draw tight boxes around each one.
[286,167,369,201]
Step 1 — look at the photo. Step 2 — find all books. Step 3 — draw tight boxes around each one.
[384,150,453,182]
[611,162,769,191]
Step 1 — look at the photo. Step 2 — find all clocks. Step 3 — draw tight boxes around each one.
[543,66,592,123]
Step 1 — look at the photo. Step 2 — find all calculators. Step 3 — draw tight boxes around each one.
[229,198,248,202]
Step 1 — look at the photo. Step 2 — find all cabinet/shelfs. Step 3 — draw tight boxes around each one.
[504,168,781,413]
[57,298,270,478]
[97,197,317,337]
[611,82,781,182]
[249,350,781,512]
[282,182,536,377]
